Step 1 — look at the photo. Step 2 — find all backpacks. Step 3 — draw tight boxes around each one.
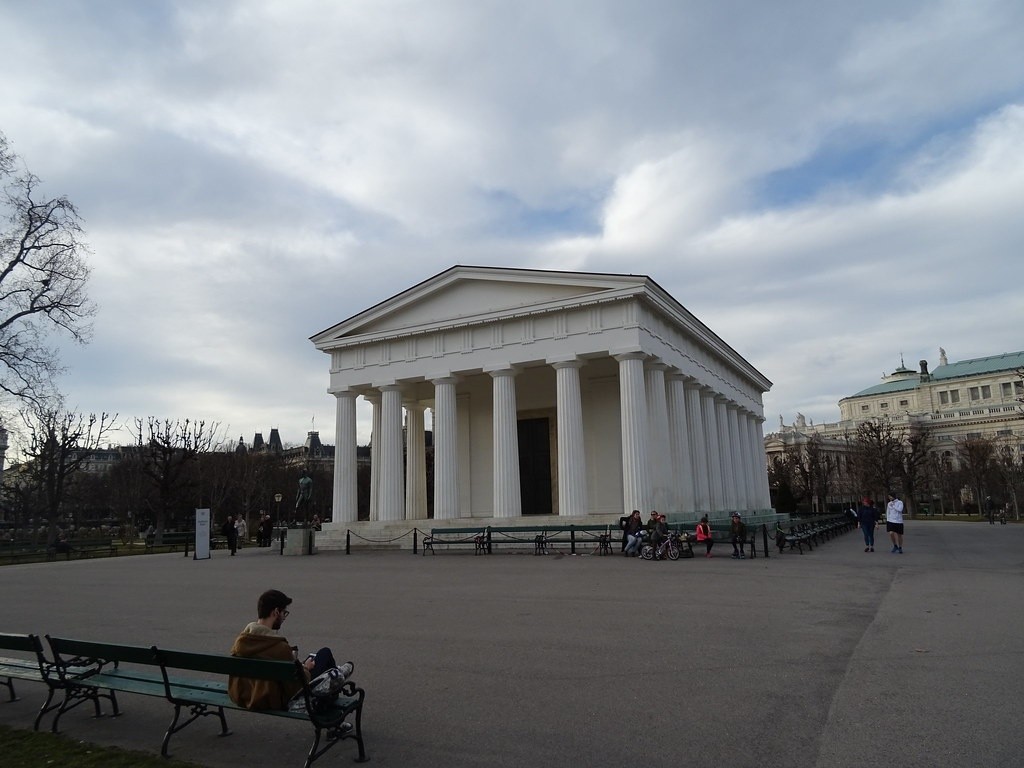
[289,668,345,716]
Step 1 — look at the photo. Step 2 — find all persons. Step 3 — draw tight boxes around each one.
[697,518,714,557]
[858,499,879,552]
[730,513,746,558]
[257,515,273,547]
[311,515,321,530]
[984,496,995,524]
[227,589,354,741]
[887,492,903,553]
[620,510,643,557]
[647,511,671,561]
[999,510,1006,525]
[289,471,312,526]
[225,515,247,556]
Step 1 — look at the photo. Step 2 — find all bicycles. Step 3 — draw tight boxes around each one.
[640,535,680,560]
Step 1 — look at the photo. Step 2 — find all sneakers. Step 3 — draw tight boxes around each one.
[338,661,354,679]
[323,722,353,741]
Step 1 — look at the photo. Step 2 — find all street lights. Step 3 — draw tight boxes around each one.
[275,493,282,541]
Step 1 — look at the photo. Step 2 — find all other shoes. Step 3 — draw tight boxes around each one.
[891,545,898,552]
[652,556,658,561]
[732,551,739,556]
[870,548,874,552]
[740,551,745,558]
[864,548,869,552]
[705,552,713,558]
[660,555,667,560]
[898,546,903,554]
[631,552,636,557]
[624,551,628,557]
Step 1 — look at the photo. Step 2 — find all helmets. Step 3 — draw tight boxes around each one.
[732,512,740,518]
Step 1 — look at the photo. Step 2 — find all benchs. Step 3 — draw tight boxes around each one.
[538,525,608,557]
[0,542,16,565]
[213,540,245,550]
[0,631,105,733]
[67,539,118,561]
[678,524,757,559]
[145,538,178,553]
[775,515,857,555]
[11,541,51,564]
[153,643,372,768]
[43,634,234,760]
[423,527,486,556]
[478,526,545,556]
[604,524,679,559]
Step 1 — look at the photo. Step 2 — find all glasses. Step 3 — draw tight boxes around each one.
[651,514,657,516]
[278,609,289,617]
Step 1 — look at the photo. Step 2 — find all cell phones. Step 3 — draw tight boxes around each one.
[301,654,316,665]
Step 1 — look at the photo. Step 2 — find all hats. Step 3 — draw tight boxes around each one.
[863,497,871,504]
